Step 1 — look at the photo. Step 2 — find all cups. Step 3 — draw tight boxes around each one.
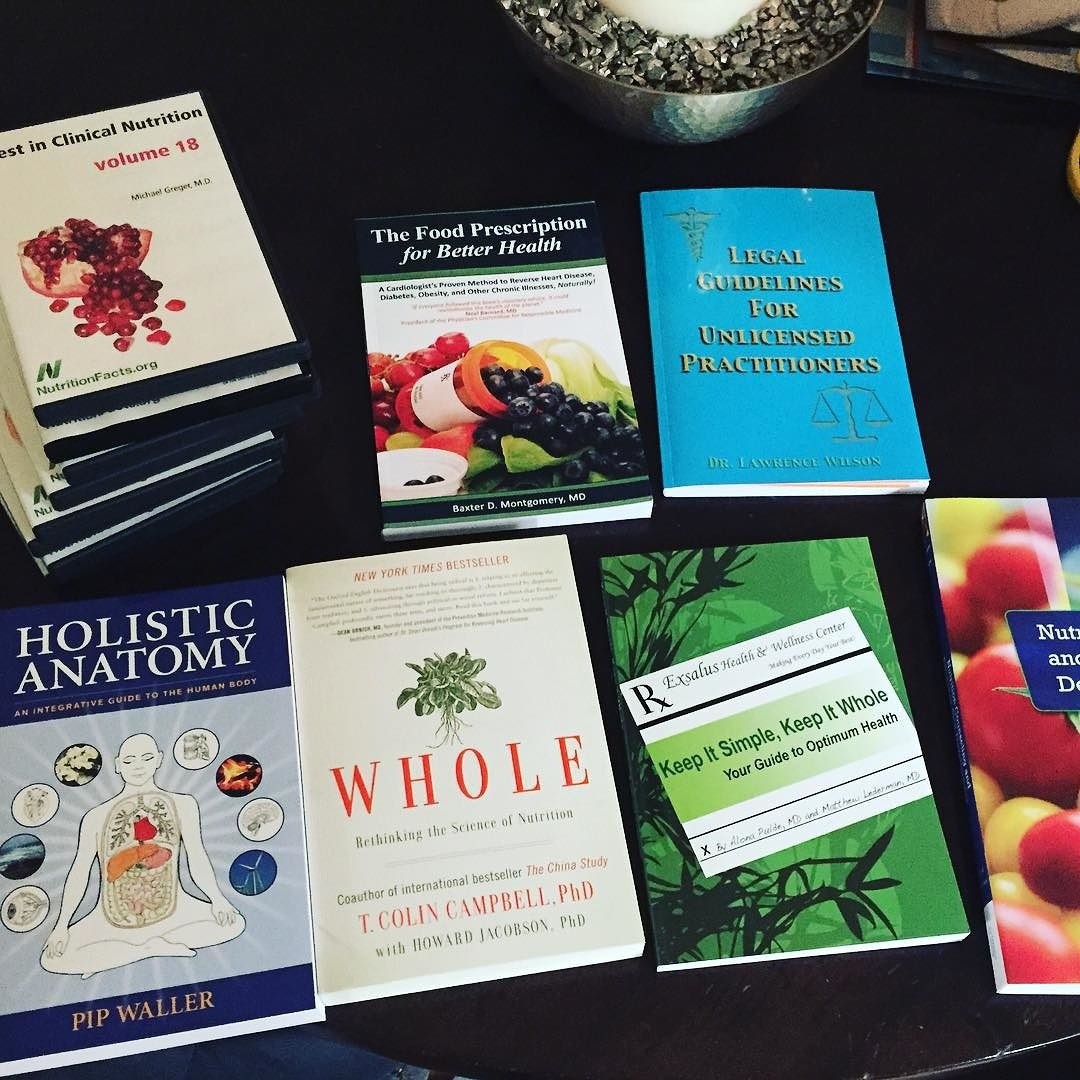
[395,341,552,438]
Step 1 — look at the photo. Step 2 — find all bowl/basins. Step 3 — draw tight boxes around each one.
[494,1,885,146]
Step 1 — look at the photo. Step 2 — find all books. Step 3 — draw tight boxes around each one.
[640,188,931,497]
[362,257,654,538]
[0,91,304,578]
[288,533,646,1009]
[923,499,1080,996]
[601,534,970,972]
[0,579,324,1076]
[865,0,1080,103]
